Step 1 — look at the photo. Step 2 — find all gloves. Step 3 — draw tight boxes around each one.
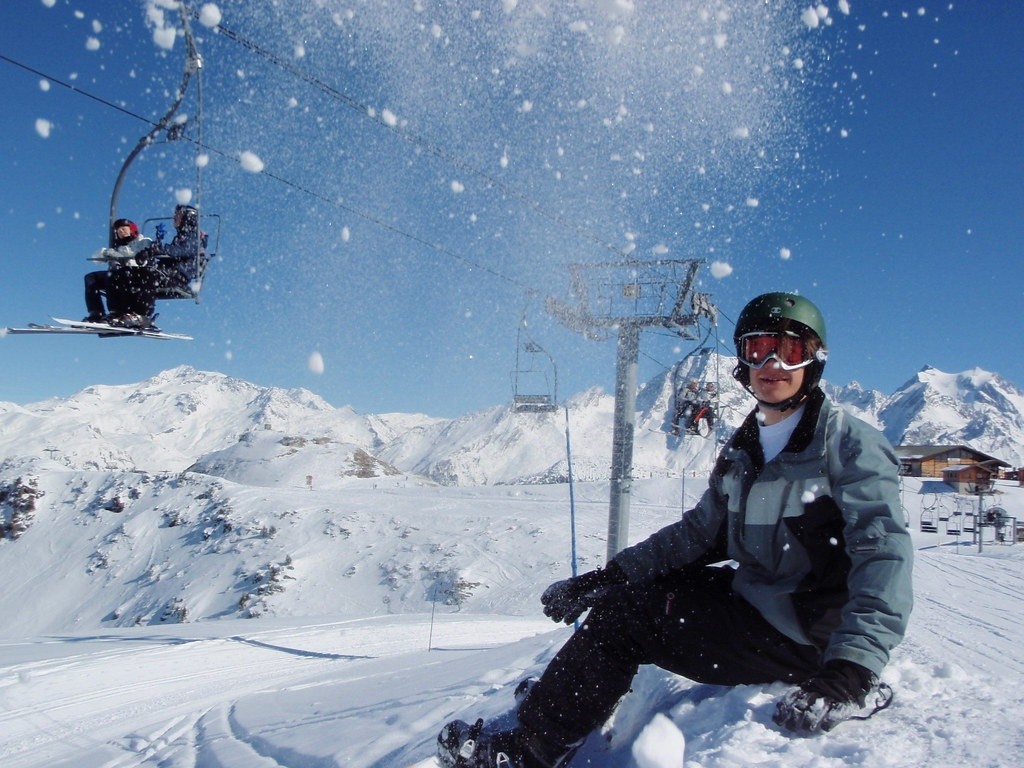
[771,661,878,739]
[90,247,106,264]
[541,565,625,626]
[134,245,154,266]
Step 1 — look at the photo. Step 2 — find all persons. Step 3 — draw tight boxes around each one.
[82,205,204,328]
[672,377,718,436]
[436,293,914,768]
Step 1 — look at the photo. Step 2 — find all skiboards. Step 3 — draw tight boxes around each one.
[3,315,195,344]
[649,423,710,442]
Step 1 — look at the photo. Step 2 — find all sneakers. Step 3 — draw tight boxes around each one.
[436,718,584,768]
[112,312,150,328]
[514,676,588,746]
[71,314,107,328]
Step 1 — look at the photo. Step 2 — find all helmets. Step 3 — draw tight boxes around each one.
[112,218,138,241]
[733,292,827,388]
[174,203,197,232]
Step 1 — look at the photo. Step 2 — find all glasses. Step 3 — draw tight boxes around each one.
[737,332,816,371]
